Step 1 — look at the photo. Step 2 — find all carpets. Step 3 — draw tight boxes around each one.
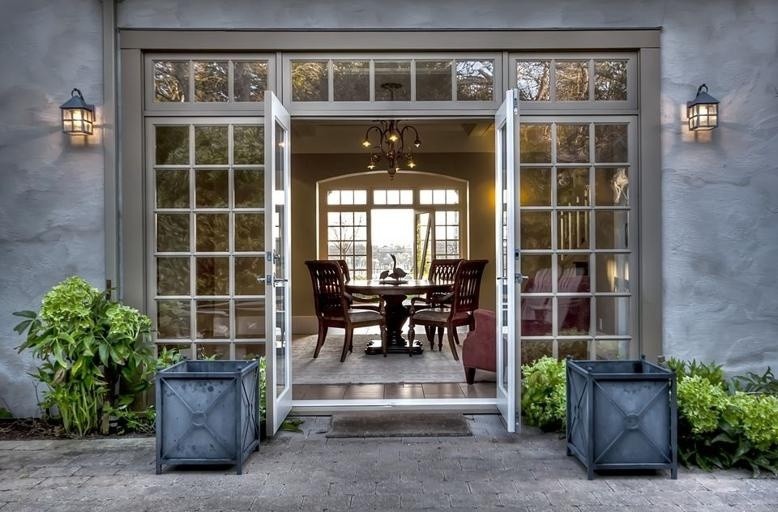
[324,413,473,438]
[260,333,497,384]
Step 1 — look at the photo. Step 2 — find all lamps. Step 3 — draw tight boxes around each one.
[58,88,95,138]
[359,83,421,177]
[686,82,720,132]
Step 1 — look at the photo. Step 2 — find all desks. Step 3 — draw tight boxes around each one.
[327,279,457,355]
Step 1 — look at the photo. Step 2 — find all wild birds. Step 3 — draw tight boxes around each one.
[379,253,407,281]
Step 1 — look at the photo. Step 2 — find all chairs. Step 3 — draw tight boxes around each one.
[462,265,588,384]
[304,259,387,363]
[408,259,489,361]
